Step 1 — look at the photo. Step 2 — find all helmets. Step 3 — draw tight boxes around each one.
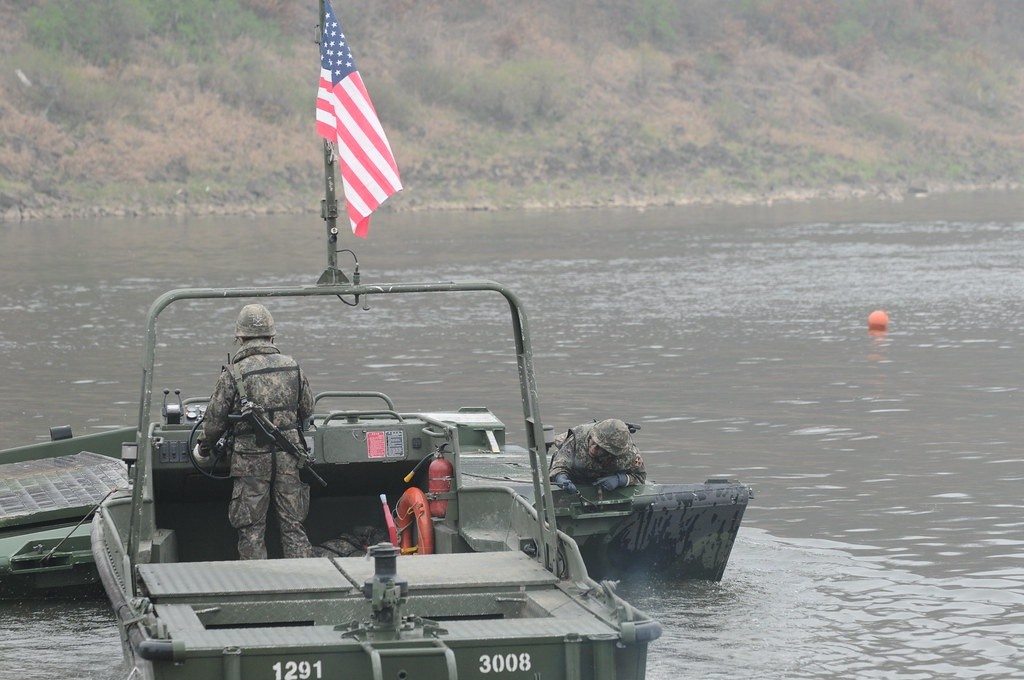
[235,304,277,336]
[589,419,628,455]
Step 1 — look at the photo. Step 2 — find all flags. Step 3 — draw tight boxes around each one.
[315,0,404,240]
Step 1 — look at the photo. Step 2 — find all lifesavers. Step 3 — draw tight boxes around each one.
[393,487,433,555]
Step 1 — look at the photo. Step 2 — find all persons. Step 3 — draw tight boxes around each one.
[192,303,315,559]
[549,419,647,495]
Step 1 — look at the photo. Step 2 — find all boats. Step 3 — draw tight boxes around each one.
[1,277,752,680]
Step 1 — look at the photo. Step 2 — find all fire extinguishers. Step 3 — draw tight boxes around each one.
[403,442,455,518]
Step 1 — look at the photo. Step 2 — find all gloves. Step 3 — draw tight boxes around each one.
[591,473,628,491]
[198,440,211,457]
[556,474,577,494]
